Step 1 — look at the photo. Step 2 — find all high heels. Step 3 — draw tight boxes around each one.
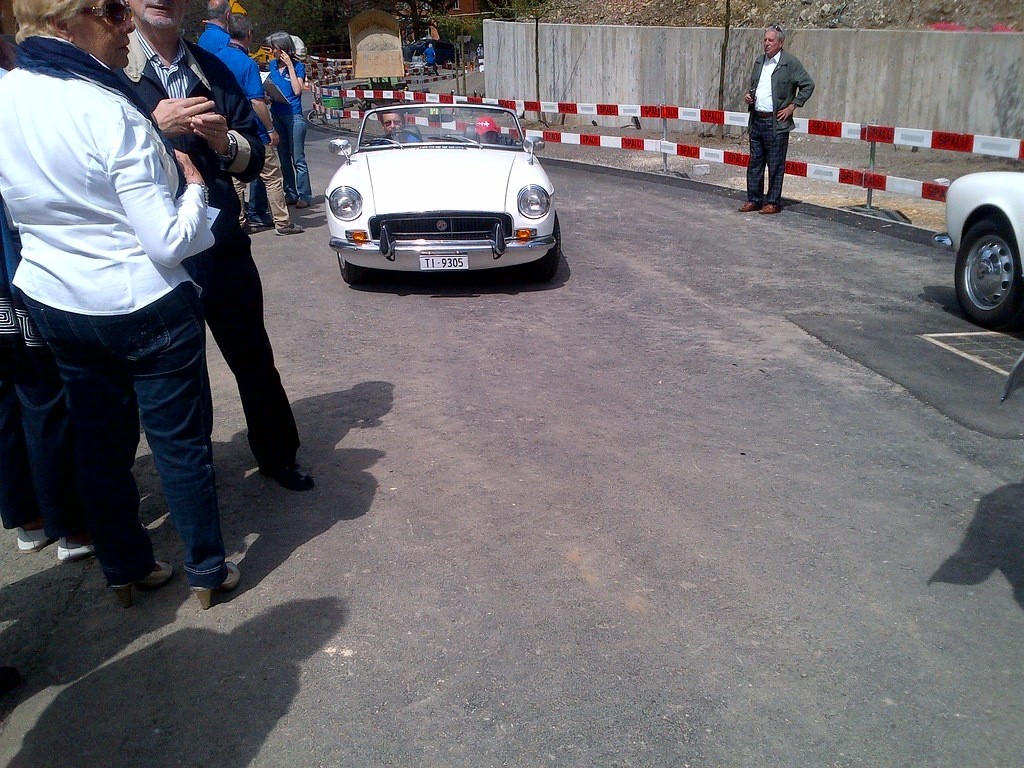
[192,561,240,610]
[110,560,173,607]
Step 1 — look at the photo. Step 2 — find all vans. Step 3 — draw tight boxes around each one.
[407,39,460,67]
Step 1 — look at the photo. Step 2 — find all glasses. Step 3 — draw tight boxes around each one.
[767,24,783,33]
[71,0,133,24]
[383,120,403,127]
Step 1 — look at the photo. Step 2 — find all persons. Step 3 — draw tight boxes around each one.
[473,116,501,145]
[0,0,316,609]
[477,44,484,66]
[371,111,421,146]
[265,32,312,209]
[423,43,439,76]
[738,29,815,215]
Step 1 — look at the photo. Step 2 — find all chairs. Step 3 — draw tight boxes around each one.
[405,125,422,138]
[464,126,477,141]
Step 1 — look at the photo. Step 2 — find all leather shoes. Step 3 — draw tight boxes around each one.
[759,203,781,214]
[739,201,762,211]
[275,462,314,491]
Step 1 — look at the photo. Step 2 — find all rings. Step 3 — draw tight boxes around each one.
[191,116,195,123]
[214,130,218,137]
[783,115,784,116]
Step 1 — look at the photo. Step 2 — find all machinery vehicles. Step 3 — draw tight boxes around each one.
[246,46,274,72]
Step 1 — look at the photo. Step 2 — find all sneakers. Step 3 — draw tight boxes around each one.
[274,224,303,235]
[241,218,256,233]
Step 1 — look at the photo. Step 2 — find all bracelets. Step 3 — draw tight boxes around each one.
[267,126,275,134]
[183,180,210,209]
[792,103,797,108]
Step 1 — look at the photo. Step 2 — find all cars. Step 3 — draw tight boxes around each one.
[931,170,1024,330]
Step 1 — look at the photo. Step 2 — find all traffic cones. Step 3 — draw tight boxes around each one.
[305,74,310,89]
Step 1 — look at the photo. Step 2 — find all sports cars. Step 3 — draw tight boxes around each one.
[325,102,561,286]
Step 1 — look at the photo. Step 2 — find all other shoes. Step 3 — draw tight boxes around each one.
[17,527,51,553]
[57,537,95,564]
[296,198,310,207]
[248,218,274,226]
[285,195,298,205]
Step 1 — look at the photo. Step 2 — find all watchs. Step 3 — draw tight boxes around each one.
[217,133,237,162]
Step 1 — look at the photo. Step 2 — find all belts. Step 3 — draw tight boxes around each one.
[755,111,773,118]
